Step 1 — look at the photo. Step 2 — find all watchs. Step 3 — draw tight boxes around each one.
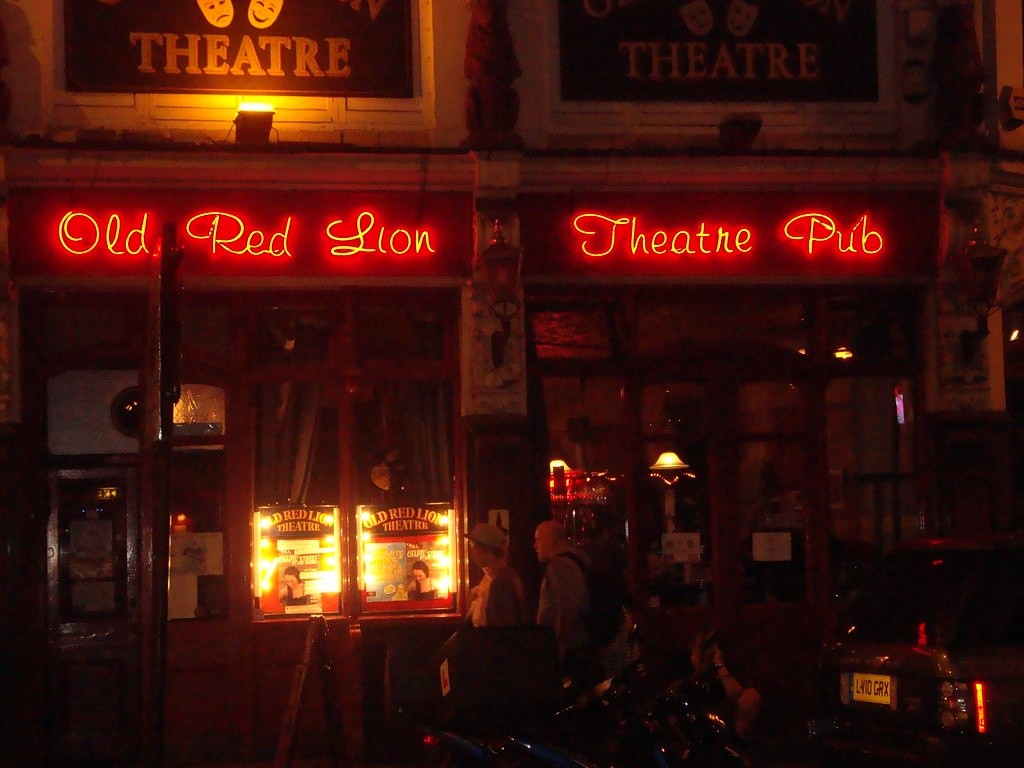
[714,661,724,672]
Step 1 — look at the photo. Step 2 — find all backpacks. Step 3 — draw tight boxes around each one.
[564,554,633,645]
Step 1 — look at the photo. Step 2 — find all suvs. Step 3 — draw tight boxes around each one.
[808,528,1024,768]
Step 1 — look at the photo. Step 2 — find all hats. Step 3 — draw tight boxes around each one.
[464,522,510,551]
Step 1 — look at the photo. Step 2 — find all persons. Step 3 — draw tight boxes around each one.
[463,521,528,624]
[533,520,593,683]
[591,578,767,768]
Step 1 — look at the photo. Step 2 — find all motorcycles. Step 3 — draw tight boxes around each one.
[411,658,760,768]
